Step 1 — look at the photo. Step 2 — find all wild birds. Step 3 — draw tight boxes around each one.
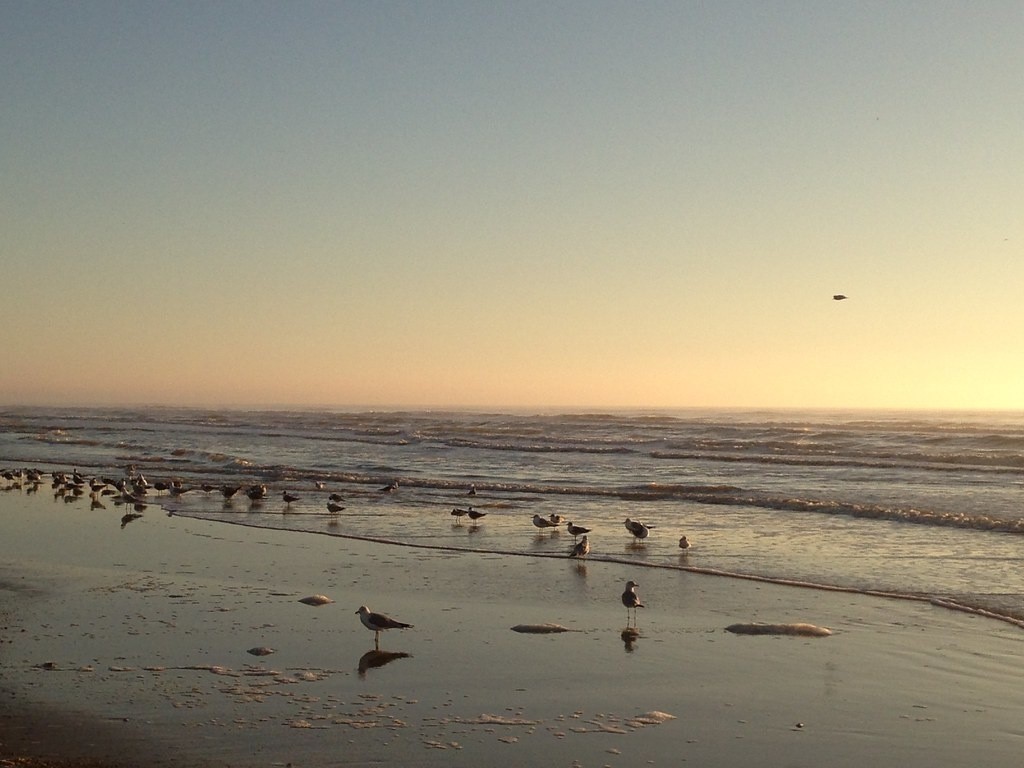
[355,605,415,642]
[621,581,644,619]
[468,487,476,495]
[377,484,397,493]
[679,536,691,551]
[280,491,297,506]
[326,494,346,518]
[531,512,592,564]
[832,295,848,301]
[0,466,267,511]
[623,518,649,542]
[451,506,488,523]
